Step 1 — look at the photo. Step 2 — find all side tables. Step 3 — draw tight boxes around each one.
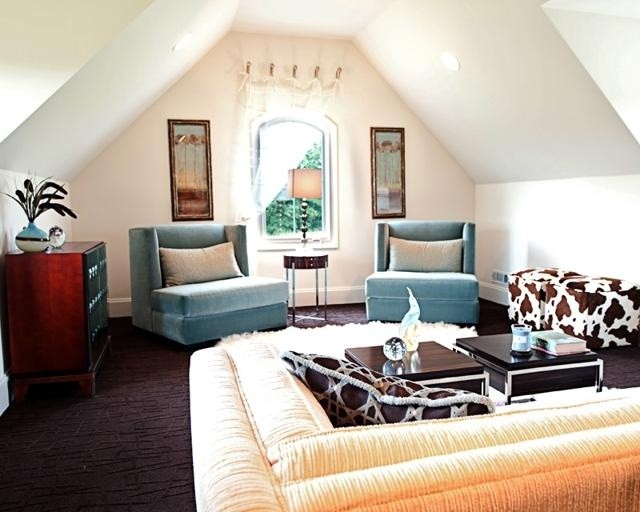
[283,253,329,325]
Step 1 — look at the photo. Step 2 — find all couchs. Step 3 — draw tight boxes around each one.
[189,339,640,512]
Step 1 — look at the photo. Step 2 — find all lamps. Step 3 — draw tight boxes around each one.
[287,170,325,253]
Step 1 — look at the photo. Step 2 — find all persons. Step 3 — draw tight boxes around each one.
[376,140,401,188]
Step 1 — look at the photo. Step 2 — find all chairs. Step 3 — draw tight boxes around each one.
[365,219,479,327]
[128,223,288,347]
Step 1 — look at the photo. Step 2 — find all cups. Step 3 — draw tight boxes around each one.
[511,323,532,352]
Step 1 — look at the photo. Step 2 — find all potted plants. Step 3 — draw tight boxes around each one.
[1,171,78,252]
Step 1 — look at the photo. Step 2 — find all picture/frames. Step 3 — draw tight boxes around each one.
[369,126,407,219]
[167,118,212,223]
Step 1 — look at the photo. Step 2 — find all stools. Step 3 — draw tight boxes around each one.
[506,267,580,333]
[547,274,639,351]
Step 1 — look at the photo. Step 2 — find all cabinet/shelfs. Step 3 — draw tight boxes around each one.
[6,240,115,405]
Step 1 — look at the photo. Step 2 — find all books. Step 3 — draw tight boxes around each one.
[531,330,590,357]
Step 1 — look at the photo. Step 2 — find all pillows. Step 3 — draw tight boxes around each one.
[159,241,245,288]
[387,238,463,273]
[284,351,494,425]
